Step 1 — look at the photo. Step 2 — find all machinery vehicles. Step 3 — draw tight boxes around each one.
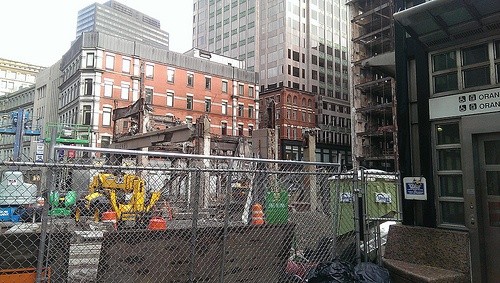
[70,175,161,226]
